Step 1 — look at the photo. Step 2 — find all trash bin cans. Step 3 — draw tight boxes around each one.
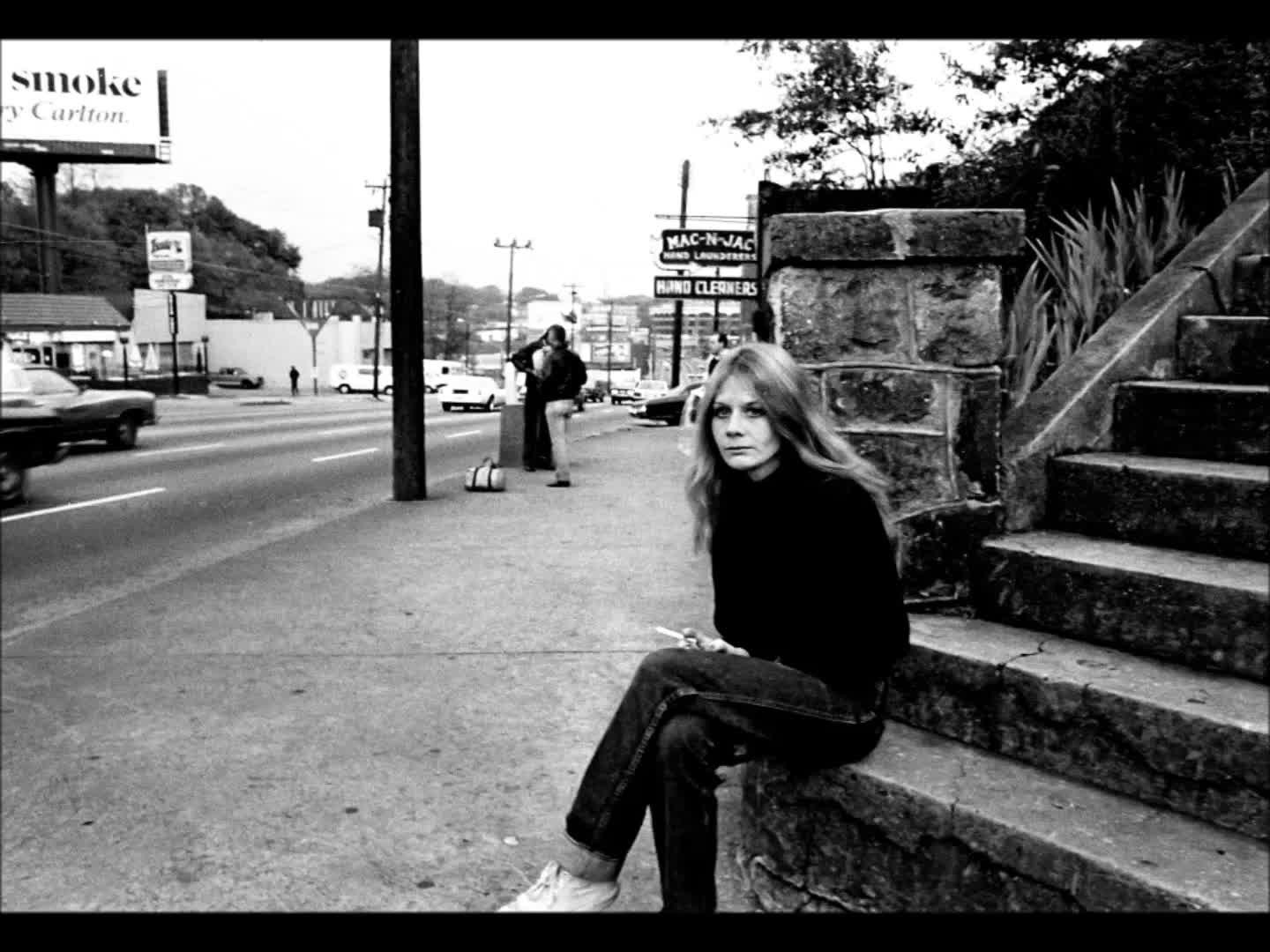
[499,404,525,469]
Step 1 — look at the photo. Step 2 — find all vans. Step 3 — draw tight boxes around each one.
[423,359,473,395]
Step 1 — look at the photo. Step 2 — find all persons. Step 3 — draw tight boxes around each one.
[539,325,588,487]
[510,333,557,472]
[707,333,728,370]
[290,367,299,395]
[498,343,912,912]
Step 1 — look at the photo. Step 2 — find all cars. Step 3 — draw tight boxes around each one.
[330,364,395,396]
[440,374,505,412]
[515,365,705,427]
[0,352,160,510]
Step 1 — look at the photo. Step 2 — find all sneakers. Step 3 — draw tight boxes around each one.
[497,860,621,912]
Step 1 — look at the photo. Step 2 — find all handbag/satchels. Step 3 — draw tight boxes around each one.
[463,458,506,493]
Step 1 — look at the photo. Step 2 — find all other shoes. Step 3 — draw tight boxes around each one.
[537,465,555,471]
[524,464,536,472]
[547,480,571,487]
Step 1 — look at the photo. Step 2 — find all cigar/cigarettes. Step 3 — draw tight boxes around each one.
[656,627,697,642]
